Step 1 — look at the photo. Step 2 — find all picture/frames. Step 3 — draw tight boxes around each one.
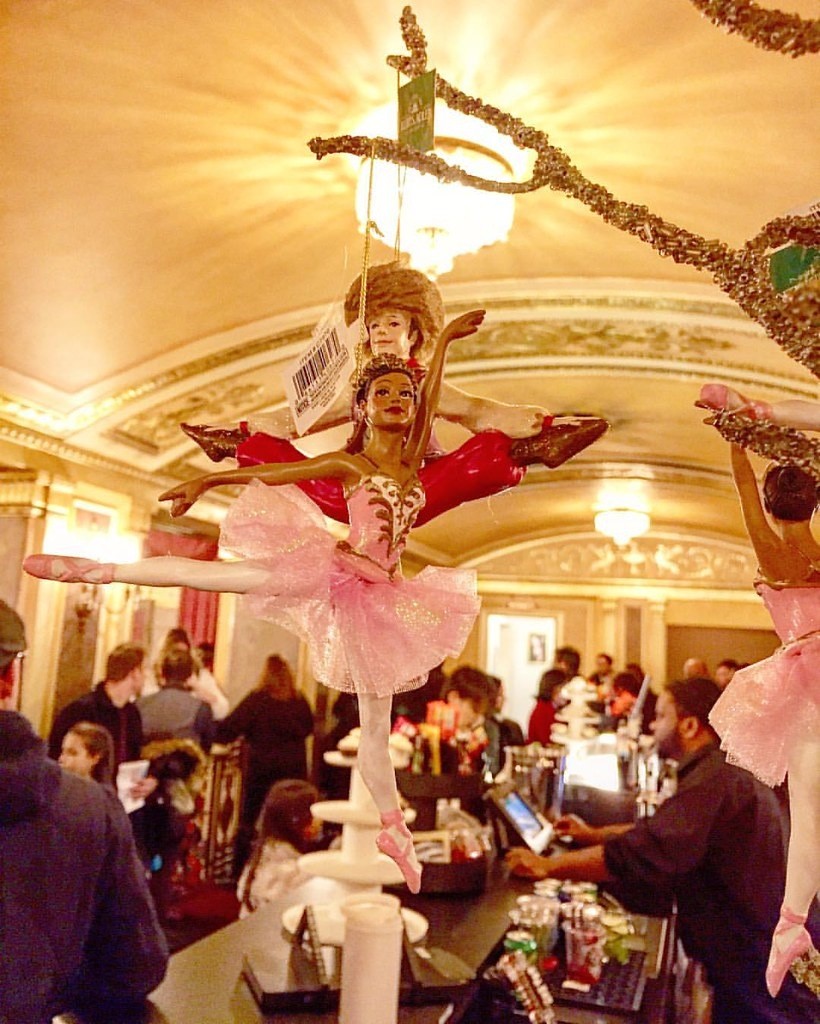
[479,609,564,741]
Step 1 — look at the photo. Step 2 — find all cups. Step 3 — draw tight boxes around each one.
[509,889,604,983]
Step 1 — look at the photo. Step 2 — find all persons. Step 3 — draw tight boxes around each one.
[503,677,820,1024]
[705,437,819,997]
[179,261,608,530]
[20,309,489,895]
[0,598,169,1023]
[0,629,818,920]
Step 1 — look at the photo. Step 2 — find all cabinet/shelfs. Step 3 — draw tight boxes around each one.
[197,742,240,888]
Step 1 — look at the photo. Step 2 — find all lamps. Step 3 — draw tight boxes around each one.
[352,102,519,279]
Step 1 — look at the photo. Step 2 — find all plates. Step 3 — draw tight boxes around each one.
[284,899,429,947]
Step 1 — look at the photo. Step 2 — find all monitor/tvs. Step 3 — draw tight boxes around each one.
[480,778,556,856]
